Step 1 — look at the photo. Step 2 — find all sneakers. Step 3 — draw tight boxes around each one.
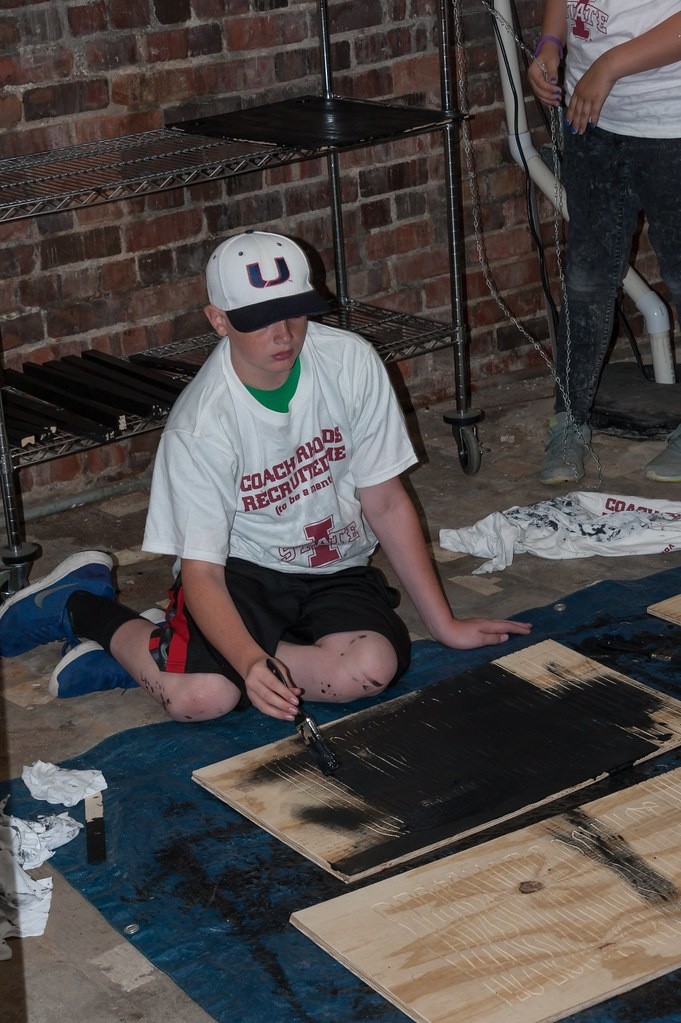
[49,609,173,698]
[646,424,681,482]
[543,412,589,483]
[0,551,115,659]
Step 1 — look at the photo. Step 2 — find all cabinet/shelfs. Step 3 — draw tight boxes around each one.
[0,0,486,606]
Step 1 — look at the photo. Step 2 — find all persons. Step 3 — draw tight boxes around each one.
[526,0,681,485]
[0,229,533,723]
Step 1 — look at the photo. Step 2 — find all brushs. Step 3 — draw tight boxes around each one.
[265,658,339,772]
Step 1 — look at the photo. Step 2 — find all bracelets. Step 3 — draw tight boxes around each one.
[534,34,564,60]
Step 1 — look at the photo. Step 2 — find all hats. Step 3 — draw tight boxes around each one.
[208,230,336,334]
